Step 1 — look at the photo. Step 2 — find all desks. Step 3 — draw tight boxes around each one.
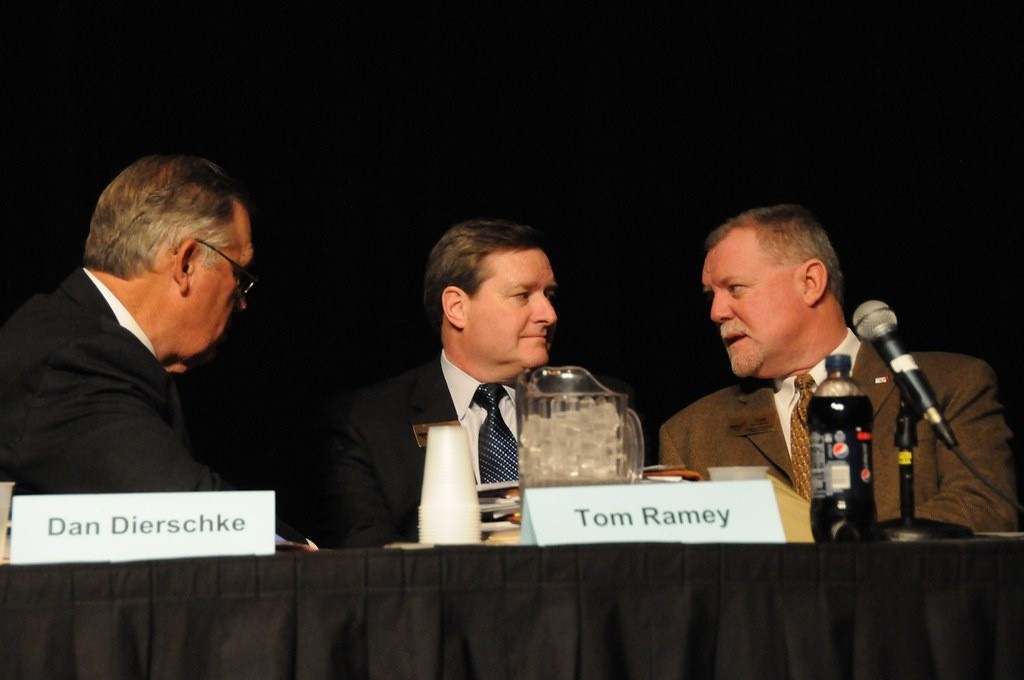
[0,537,1024,680]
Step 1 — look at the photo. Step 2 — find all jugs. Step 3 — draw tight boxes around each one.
[515,365,645,514]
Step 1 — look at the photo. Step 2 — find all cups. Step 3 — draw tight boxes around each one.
[417,425,482,545]
[0,480,16,560]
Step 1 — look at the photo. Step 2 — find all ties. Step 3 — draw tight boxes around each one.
[790,373,816,504]
[472,382,518,485]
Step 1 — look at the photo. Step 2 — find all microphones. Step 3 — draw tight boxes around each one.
[852,300,959,451]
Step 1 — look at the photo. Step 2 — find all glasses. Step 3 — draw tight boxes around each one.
[173,237,259,300]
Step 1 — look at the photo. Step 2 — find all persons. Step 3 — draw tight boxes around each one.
[0,152,314,553]
[329,220,656,547]
[659,202,1020,535]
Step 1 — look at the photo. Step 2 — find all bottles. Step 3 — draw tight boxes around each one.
[805,354,879,544]
